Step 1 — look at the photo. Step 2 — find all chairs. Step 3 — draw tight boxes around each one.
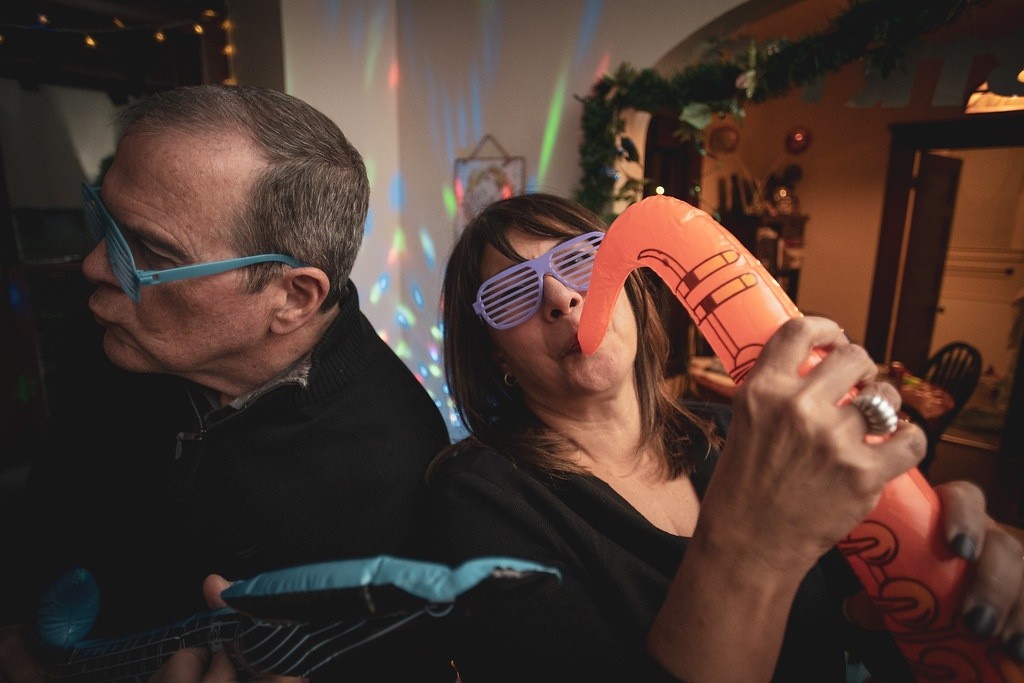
[920,340,985,467]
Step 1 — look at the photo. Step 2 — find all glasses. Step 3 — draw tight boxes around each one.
[471,230,608,330]
[77,178,313,304]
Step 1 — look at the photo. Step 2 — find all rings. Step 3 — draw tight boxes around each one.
[851,394,898,434]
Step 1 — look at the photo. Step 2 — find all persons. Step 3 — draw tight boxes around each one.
[422,191,1024,682]
[0,81,451,682]
